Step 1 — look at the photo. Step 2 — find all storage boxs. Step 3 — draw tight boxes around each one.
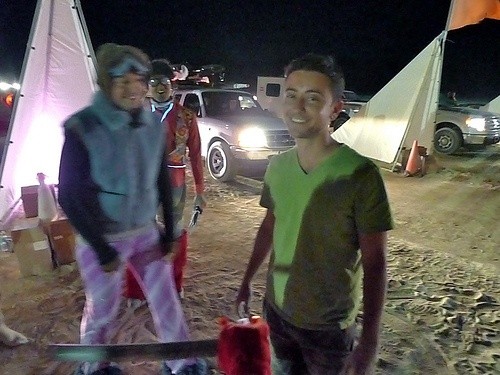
[12,218,55,276]
[21,185,56,219]
[48,218,76,266]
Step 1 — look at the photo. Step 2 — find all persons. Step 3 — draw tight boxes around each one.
[235,53,394,375]
[122,60,206,306]
[58,43,207,375]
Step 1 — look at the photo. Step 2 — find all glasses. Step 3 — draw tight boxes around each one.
[148,76,171,87]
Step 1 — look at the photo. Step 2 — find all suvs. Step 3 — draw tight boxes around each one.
[434,90,500,156]
[169,77,298,183]
[330,87,368,135]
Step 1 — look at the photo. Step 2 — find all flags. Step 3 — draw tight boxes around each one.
[449,0,500,30]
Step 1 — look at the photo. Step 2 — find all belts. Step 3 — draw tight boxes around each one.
[266,296,355,331]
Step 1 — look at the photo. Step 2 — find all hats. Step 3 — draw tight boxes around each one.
[96,43,153,91]
[147,60,175,79]
